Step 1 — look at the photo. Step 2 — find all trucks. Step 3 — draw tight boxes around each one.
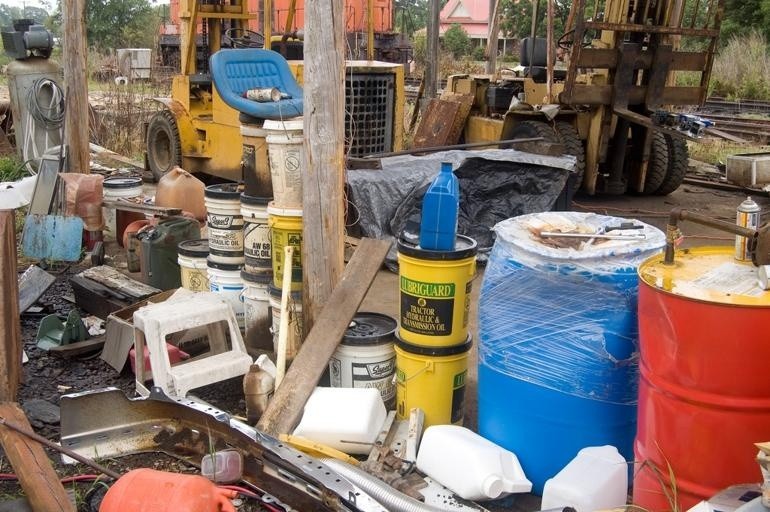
[153,0,415,75]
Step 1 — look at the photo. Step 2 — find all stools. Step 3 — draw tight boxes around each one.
[133,292,253,401]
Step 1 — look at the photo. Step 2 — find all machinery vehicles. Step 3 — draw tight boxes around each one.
[147,0,405,182]
[419,1,726,200]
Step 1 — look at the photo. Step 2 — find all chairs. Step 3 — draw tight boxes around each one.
[522,39,567,82]
[211,49,305,119]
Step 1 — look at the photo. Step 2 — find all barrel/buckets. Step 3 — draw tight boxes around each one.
[177,238,210,291]
[269,280,303,360]
[329,311,400,412]
[99,468,239,512]
[397,231,478,347]
[263,91,304,210]
[415,424,533,501]
[103,176,143,236]
[241,202,272,277]
[130,341,190,375]
[207,261,245,333]
[292,386,387,456]
[420,161,459,250]
[266,201,303,292]
[154,166,206,229]
[239,111,274,198]
[541,445,628,512]
[243,354,279,427]
[632,245,770,511]
[141,216,200,292]
[394,327,474,426]
[477,211,667,495]
[203,183,244,263]
[240,277,274,351]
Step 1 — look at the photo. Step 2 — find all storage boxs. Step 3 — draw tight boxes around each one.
[98,287,212,376]
